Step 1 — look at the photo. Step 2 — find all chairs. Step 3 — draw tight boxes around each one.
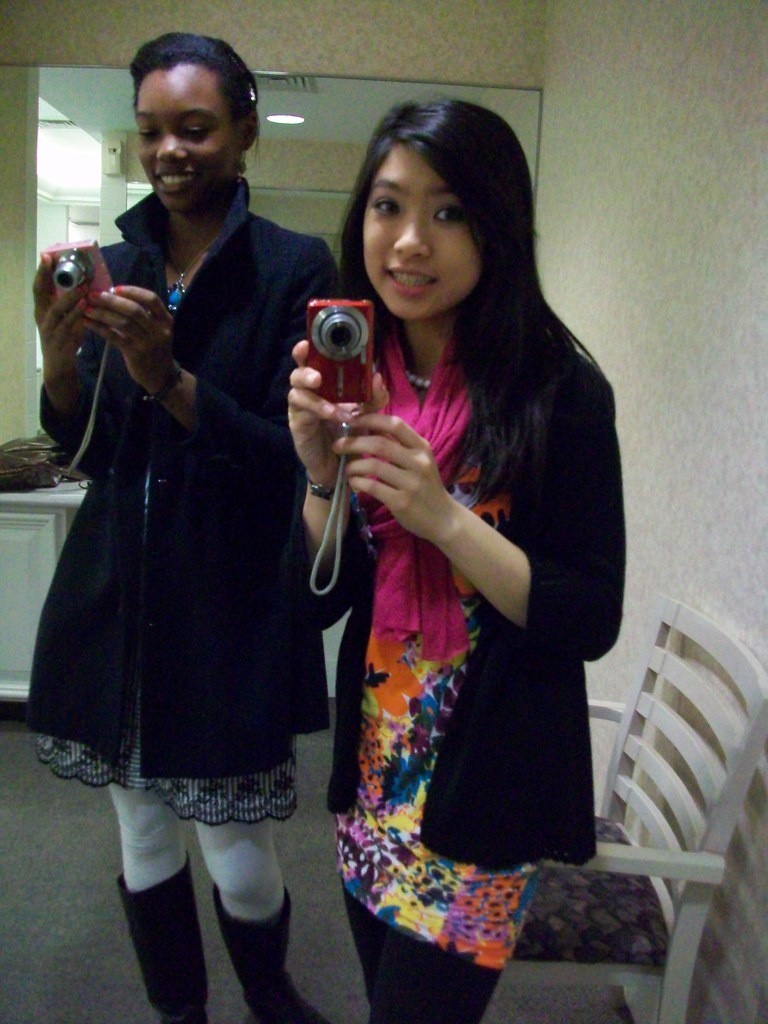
[505,595,768,1024]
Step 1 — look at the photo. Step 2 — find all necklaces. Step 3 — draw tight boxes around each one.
[164,237,218,311]
[404,368,430,389]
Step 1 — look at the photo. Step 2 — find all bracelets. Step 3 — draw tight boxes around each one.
[306,471,349,499]
[136,355,180,404]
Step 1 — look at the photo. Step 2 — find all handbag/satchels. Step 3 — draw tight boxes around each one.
[0,430,89,489]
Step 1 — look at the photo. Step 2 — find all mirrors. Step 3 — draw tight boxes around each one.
[33,64,542,440]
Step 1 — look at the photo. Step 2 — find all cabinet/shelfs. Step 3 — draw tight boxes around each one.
[0,510,84,701]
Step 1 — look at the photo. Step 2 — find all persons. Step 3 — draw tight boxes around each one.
[283,100,625,1024]
[30,32,339,1024]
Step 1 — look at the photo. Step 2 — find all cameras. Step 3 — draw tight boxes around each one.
[306,298,374,406]
[41,239,118,316]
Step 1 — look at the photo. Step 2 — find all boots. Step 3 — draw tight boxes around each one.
[213,882,332,1024]
[117,850,209,1024]
